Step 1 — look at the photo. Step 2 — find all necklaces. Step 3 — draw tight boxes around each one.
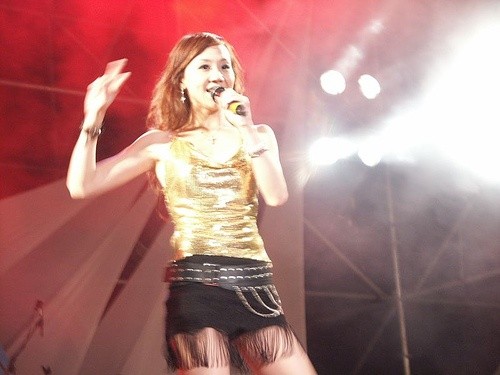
[188,122,224,145]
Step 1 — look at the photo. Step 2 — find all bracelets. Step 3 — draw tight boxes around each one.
[79,122,101,137]
[247,147,267,158]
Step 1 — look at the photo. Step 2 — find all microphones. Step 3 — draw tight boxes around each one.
[211,84,246,116]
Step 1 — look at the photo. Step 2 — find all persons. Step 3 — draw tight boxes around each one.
[65,31,318,375]
